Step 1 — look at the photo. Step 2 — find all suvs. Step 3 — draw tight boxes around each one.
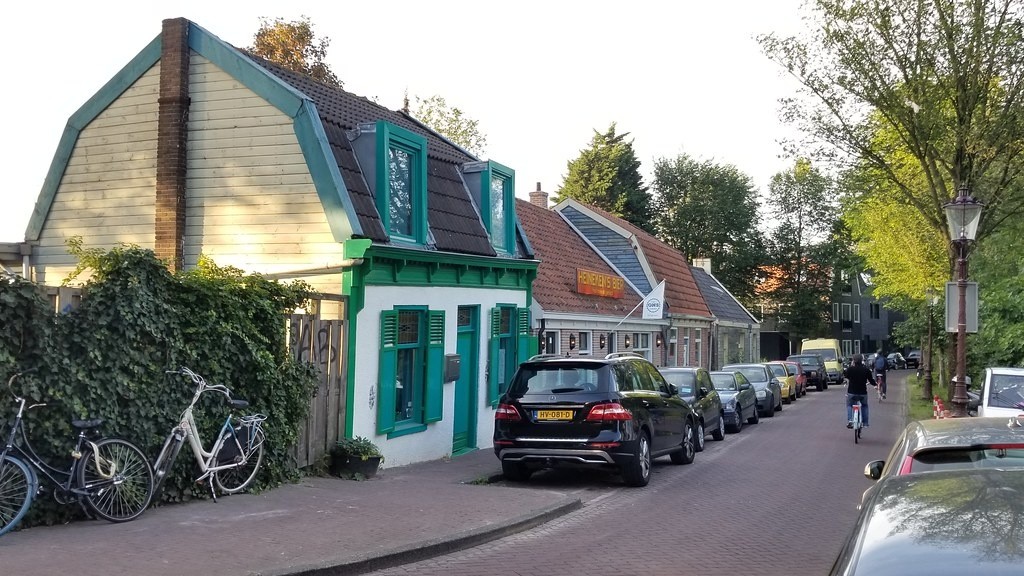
[492,352,698,489]
[786,353,828,392]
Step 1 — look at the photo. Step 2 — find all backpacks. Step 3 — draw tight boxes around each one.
[876,356,886,369]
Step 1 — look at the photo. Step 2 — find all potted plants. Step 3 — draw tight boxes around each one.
[333,434,385,479]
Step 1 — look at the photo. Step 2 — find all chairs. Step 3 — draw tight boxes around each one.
[562,369,580,388]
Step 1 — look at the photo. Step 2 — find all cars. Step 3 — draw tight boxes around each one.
[843,349,922,373]
[653,366,725,453]
[720,364,783,418]
[828,415,1024,576]
[969,367,1024,419]
[762,362,797,405]
[771,362,807,398]
[708,371,759,434]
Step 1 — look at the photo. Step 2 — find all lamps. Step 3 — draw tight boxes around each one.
[570,333,576,350]
[625,334,630,348]
[657,334,660,347]
[541,335,546,350]
[600,334,605,349]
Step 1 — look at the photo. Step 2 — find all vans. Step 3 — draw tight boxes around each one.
[800,337,844,384]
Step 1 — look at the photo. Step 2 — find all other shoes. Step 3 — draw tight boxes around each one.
[882,394,886,399]
[874,386,877,389]
[862,424,869,428]
[847,422,852,429]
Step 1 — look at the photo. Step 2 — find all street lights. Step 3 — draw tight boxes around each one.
[938,181,987,417]
[921,287,938,400]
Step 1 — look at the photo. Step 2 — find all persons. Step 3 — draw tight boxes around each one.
[843,354,877,429]
[874,349,890,399]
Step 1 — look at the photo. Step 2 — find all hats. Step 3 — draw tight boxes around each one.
[852,354,863,363]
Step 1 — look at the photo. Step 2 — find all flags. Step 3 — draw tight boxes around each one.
[643,281,665,319]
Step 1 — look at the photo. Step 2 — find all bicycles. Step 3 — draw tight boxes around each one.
[843,380,871,443]
[0,368,155,537]
[871,369,885,404]
[145,358,267,512]
